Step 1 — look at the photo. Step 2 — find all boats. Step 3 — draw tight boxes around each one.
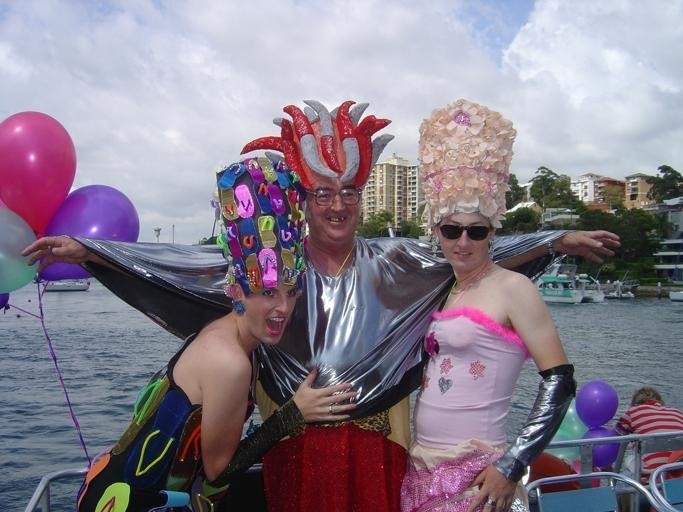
[601,277,640,298]
[669,290,683,301]
[43,280,90,292]
[535,274,604,305]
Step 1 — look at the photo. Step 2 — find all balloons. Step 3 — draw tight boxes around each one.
[40,184,140,283]
[0,208,41,294]
[543,380,621,486]
[0,293,10,310]
[0,111,77,236]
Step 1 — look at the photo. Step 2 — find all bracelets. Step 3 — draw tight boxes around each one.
[548,242,553,255]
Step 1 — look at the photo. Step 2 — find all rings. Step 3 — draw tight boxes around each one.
[487,498,496,506]
[329,404,332,414]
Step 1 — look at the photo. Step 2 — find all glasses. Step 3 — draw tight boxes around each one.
[438,224,492,240]
[304,187,363,207]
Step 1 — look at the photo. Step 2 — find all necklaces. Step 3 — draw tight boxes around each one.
[451,271,490,294]
[315,246,355,278]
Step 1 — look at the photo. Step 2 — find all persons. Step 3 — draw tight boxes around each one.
[76,152,360,511]
[614,387,683,485]
[401,99,578,512]
[20,100,622,512]
